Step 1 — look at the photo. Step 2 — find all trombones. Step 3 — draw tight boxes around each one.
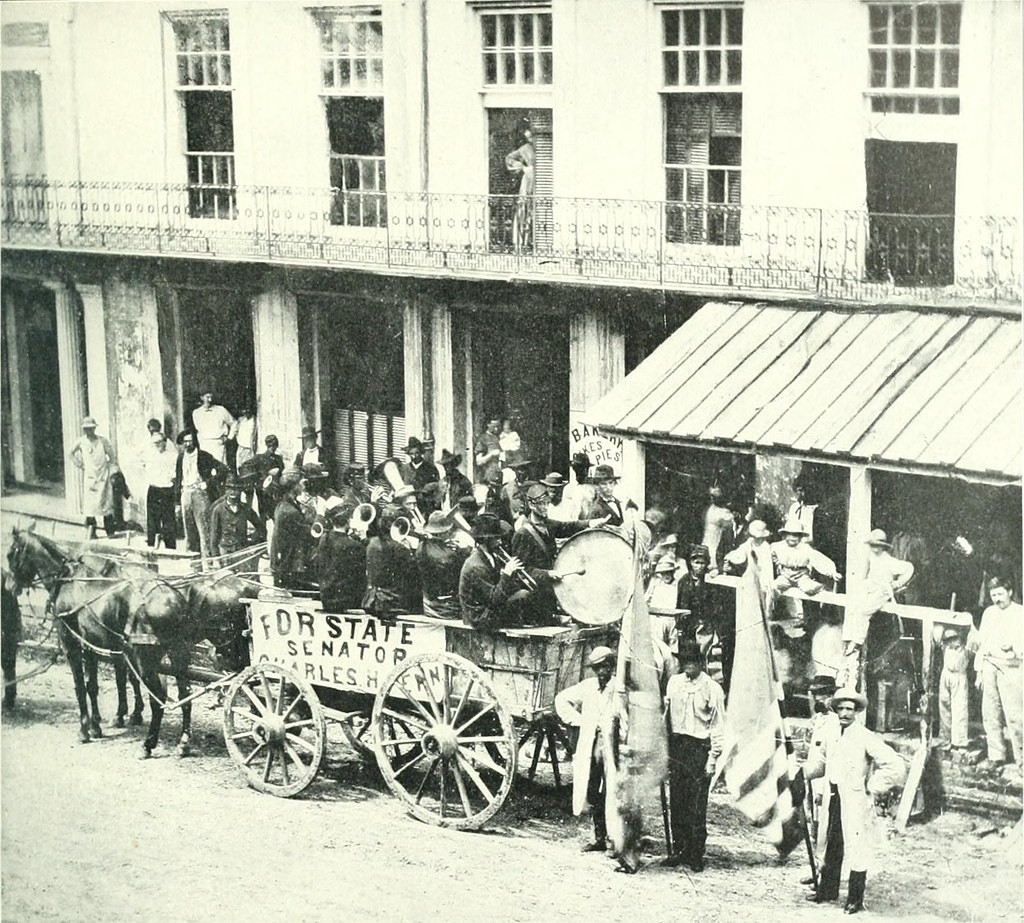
[352,503,377,527]
[372,455,429,527]
[446,503,540,594]
[389,518,445,545]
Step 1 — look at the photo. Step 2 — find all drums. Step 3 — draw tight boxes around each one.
[550,526,655,629]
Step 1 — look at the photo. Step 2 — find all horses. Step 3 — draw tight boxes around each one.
[3,520,258,759]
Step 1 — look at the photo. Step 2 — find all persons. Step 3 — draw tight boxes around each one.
[664,644,724,874]
[554,647,614,854]
[932,575,1023,775]
[646,487,843,688]
[134,389,623,635]
[845,528,914,655]
[506,128,535,253]
[796,674,902,914]
[70,418,124,539]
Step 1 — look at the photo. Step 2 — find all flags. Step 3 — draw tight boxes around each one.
[716,569,806,853]
[607,546,671,791]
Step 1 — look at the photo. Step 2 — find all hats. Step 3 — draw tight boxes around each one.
[297,425,323,438]
[748,520,770,538]
[527,485,549,501]
[943,628,962,643]
[540,472,568,486]
[80,417,98,429]
[690,545,711,564]
[177,428,197,444]
[864,529,893,550]
[655,557,680,573]
[224,473,244,488]
[809,676,838,692]
[672,643,706,662]
[402,437,426,455]
[426,511,453,534]
[592,465,620,480]
[470,514,511,539]
[590,646,613,665]
[778,520,810,538]
[437,449,461,464]
[663,535,679,545]
[831,689,869,708]
[569,453,594,467]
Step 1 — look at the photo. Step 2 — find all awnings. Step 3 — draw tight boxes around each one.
[576,303,1023,485]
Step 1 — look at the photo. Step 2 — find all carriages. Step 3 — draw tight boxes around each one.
[4,517,622,830]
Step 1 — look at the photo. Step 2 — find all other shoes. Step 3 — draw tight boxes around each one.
[844,903,861,916]
[806,893,820,901]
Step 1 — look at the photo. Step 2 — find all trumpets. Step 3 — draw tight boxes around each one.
[310,521,330,538]
